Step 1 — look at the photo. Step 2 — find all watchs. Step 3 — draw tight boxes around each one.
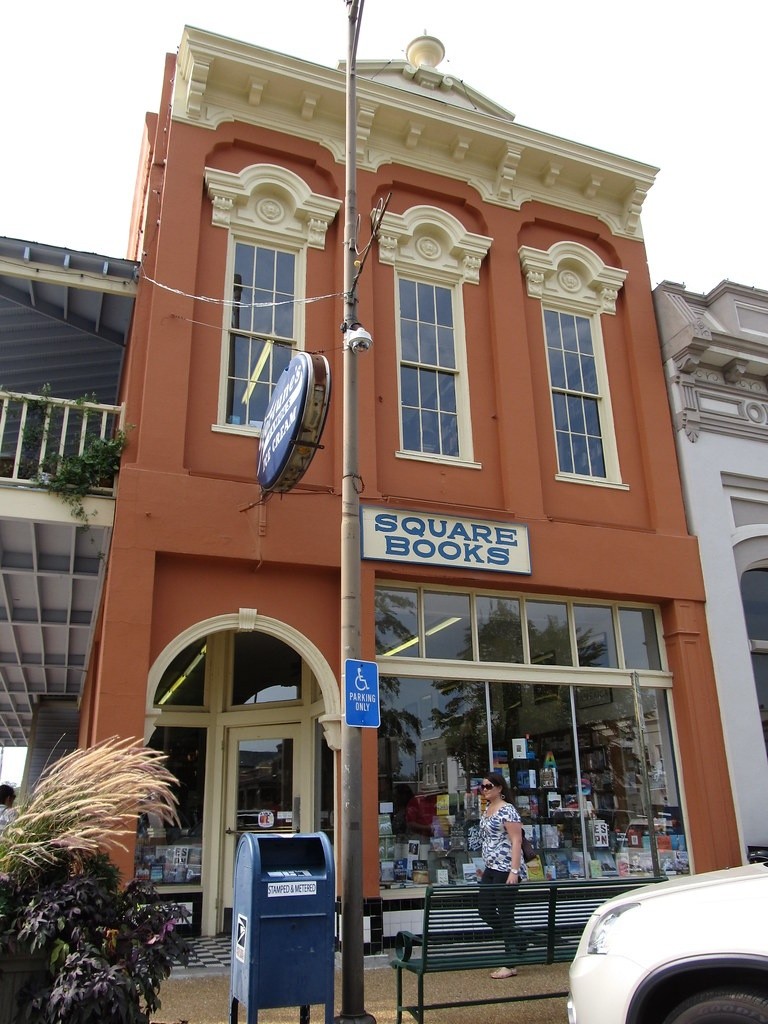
[511,869,519,875]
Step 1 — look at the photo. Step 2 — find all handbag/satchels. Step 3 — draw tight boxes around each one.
[521,828,536,863]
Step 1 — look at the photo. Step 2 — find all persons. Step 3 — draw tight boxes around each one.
[0,784,20,831]
[478,771,530,979]
[405,772,442,844]
[391,784,434,843]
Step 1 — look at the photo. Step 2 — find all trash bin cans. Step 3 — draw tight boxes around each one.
[227,829,336,1024]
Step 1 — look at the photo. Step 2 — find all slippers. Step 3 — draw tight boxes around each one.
[490,967,517,978]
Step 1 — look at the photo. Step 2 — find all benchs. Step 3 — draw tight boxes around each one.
[388,877,669,1024]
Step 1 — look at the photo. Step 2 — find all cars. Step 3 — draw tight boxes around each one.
[564,862,768,1023]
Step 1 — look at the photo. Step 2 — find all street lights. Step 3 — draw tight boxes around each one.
[335,0,373,1017]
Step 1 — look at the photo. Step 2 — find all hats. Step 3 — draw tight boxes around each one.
[420,774,440,793]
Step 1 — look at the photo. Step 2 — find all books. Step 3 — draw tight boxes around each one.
[378,795,486,885]
[134,827,201,884]
[513,738,691,884]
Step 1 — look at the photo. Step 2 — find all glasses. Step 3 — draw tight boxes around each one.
[481,784,495,790]
[11,794,16,799]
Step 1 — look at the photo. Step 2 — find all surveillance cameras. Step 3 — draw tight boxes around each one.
[347,327,374,355]
